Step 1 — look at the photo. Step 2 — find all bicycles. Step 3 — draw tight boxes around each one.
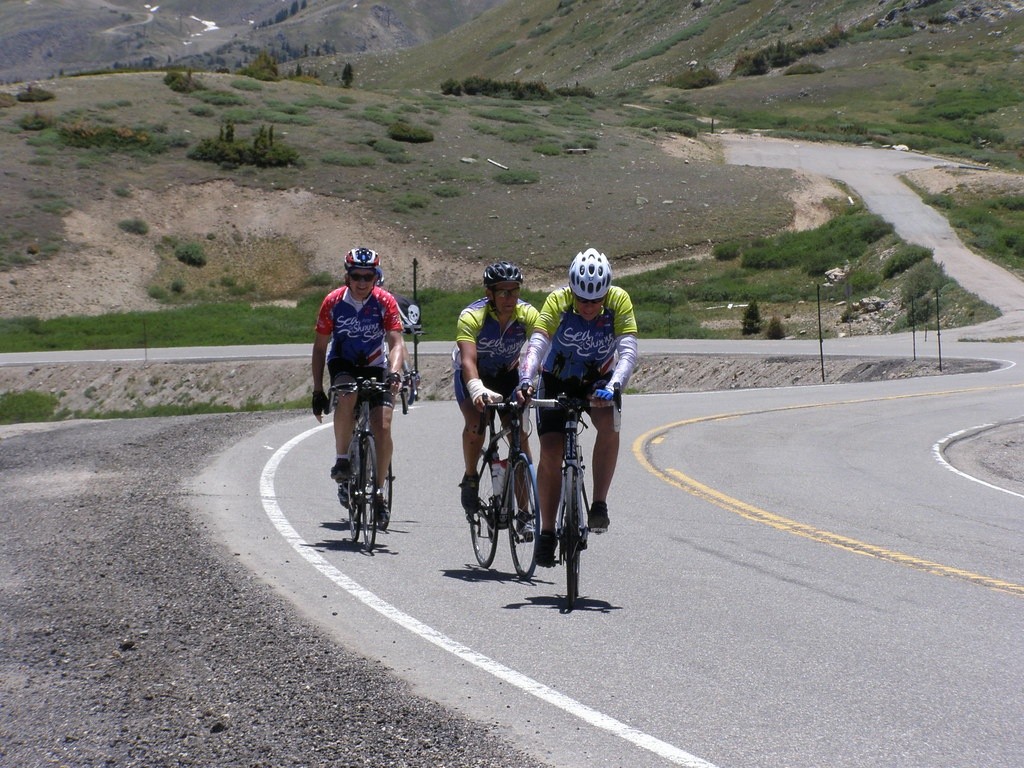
[465,381,576,581]
[513,382,625,601]
[327,375,413,554]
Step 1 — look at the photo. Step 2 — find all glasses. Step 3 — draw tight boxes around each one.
[491,287,520,298]
[350,273,375,281]
[578,299,602,304]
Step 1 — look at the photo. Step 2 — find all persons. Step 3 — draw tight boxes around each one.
[311,246,413,530]
[513,247,638,567]
[454,260,539,532]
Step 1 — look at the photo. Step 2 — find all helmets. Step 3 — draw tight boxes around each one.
[344,248,380,270]
[482,261,523,285]
[569,248,612,300]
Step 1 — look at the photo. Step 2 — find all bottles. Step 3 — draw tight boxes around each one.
[492,452,501,495]
[498,459,508,495]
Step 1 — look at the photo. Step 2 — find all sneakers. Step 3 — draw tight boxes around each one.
[589,501,609,533]
[331,458,351,479]
[517,512,532,542]
[376,499,389,530]
[534,531,558,567]
[459,471,479,512]
